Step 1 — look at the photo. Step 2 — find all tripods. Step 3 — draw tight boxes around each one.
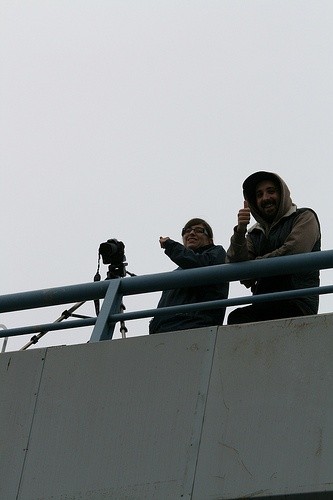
[19,263,128,350]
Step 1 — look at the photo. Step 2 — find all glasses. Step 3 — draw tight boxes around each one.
[183,227,208,237]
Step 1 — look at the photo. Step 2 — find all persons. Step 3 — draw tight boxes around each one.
[148,218,230,334]
[224,171,322,325]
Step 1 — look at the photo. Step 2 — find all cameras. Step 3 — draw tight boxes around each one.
[100,238,126,265]
